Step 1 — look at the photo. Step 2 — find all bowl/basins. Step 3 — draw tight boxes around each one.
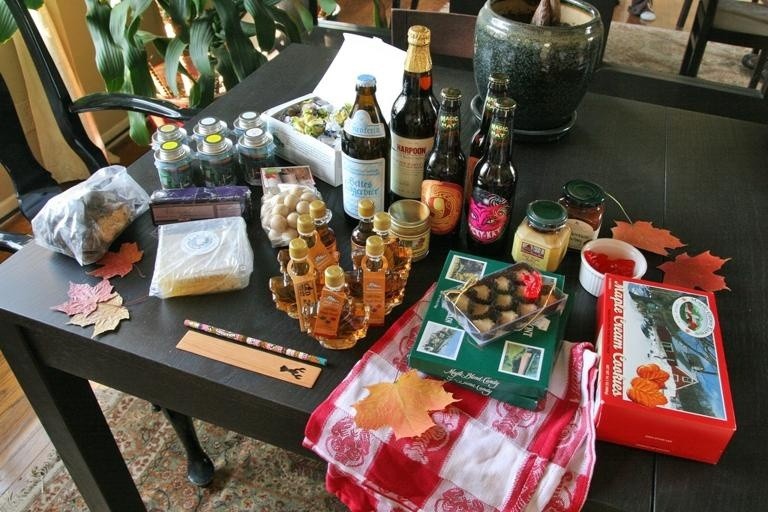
[578,237,648,297]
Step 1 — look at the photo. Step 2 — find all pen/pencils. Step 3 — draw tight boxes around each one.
[184,319,328,367]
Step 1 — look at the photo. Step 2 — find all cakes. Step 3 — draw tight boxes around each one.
[437,263,568,342]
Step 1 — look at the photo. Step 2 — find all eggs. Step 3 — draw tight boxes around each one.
[260,186,321,246]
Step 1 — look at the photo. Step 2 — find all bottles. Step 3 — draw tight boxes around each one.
[466,97,528,259]
[302,265,370,351]
[154,140,195,190]
[344,239,410,316]
[351,198,375,270]
[268,237,324,320]
[309,199,336,252]
[150,124,190,151]
[276,213,340,287]
[193,116,230,145]
[341,74,389,224]
[420,87,466,245]
[351,211,412,274]
[388,25,440,213]
[234,127,278,186]
[463,73,514,214]
[230,110,268,144]
[195,133,240,189]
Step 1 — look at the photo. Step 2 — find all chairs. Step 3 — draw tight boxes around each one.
[675,0,768,89]
[0,0,217,489]
[390,7,478,59]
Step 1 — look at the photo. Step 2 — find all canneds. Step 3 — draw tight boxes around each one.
[510,200,571,273]
[385,200,433,262]
[558,180,606,255]
